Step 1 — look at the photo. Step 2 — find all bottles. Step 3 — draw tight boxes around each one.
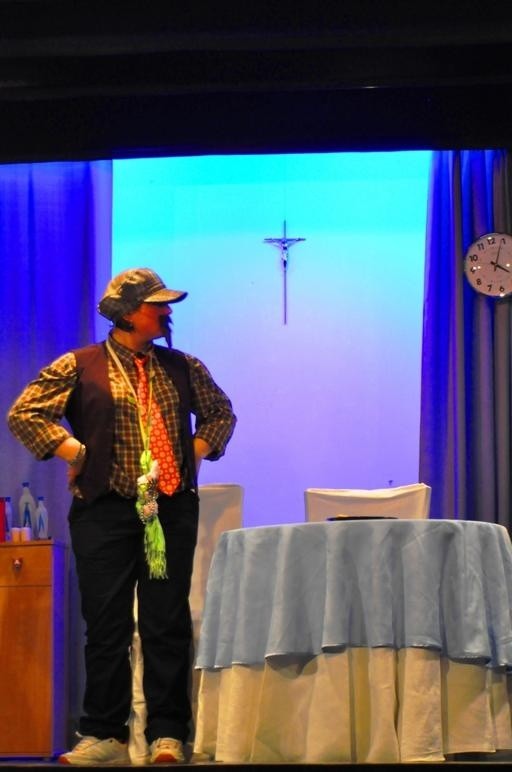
[10,527,32,544]
[0,496,14,544]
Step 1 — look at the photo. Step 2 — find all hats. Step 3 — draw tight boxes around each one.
[96,268,188,326]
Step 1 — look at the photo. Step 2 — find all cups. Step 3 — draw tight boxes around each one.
[19,482,49,540]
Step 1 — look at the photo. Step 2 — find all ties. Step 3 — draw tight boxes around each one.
[131,355,182,496]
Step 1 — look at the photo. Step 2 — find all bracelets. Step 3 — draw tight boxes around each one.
[69,442,85,467]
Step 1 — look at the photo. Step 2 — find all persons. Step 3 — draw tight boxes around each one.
[5,266,242,768]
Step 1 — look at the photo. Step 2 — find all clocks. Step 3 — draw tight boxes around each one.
[464,231,512,299]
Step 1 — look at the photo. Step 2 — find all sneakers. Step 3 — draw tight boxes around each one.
[57,731,133,767]
[149,736,187,764]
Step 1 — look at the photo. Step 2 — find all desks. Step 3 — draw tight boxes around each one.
[220,518,505,766]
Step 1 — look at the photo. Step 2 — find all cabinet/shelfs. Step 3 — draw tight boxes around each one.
[2,539,69,763]
